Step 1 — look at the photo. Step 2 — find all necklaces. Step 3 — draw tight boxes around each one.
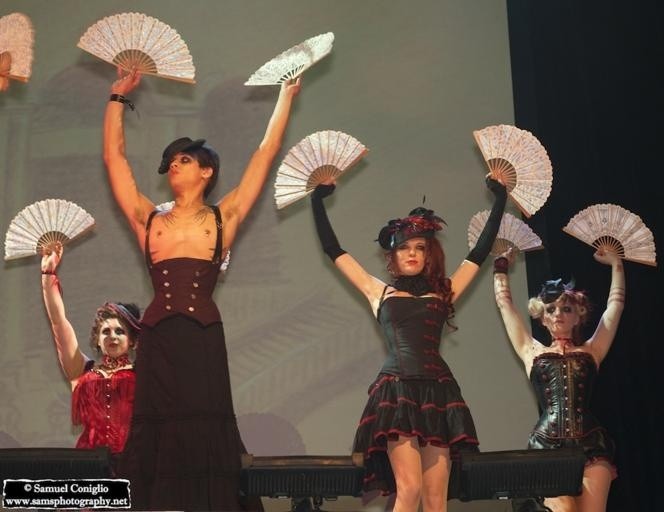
[103,369,115,376]
[551,337,575,344]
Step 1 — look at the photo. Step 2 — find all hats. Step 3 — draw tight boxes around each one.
[158,137,205,174]
[379,207,436,251]
[539,277,566,304]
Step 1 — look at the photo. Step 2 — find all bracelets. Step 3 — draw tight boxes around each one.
[493,257,509,274]
[108,94,135,111]
[40,270,64,276]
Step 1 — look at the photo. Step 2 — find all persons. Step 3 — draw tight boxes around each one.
[493,245,626,512]
[104,59,301,511]
[311,173,506,511]
[40,242,141,478]
